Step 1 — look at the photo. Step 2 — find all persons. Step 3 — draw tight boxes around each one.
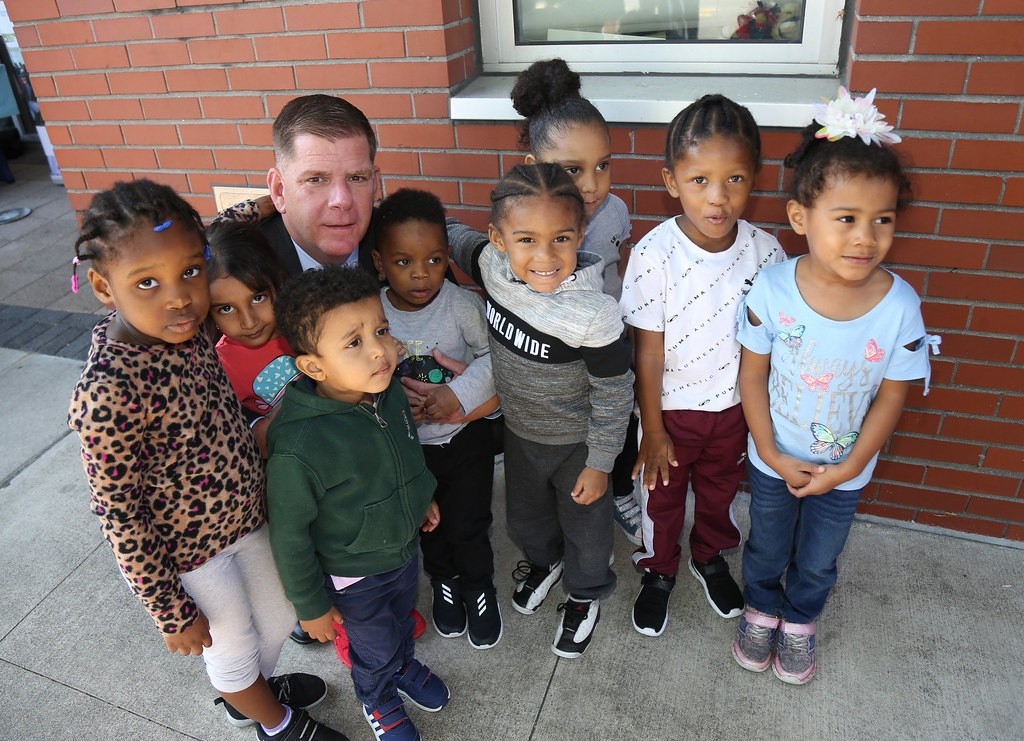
[66,178,353,741]
[731,116,941,686]
[509,58,644,565]
[618,93,788,637]
[264,264,450,741]
[445,161,618,659]
[209,93,501,669]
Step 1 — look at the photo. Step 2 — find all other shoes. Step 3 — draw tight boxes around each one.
[330,617,353,670]
[411,607,426,638]
[289,621,318,645]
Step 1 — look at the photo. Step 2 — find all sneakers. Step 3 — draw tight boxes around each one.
[550,591,600,659]
[511,557,565,616]
[631,566,677,636]
[362,690,422,741]
[255,701,350,741]
[731,601,781,673]
[430,572,468,639]
[467,572,504,649]
[771,614,817,686]
[395,657,451,712]
[688,550,745,618]
[609,490,644,565]
[214,672,328,728]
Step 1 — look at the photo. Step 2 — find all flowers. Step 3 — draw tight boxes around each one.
[809,84,902,147]
